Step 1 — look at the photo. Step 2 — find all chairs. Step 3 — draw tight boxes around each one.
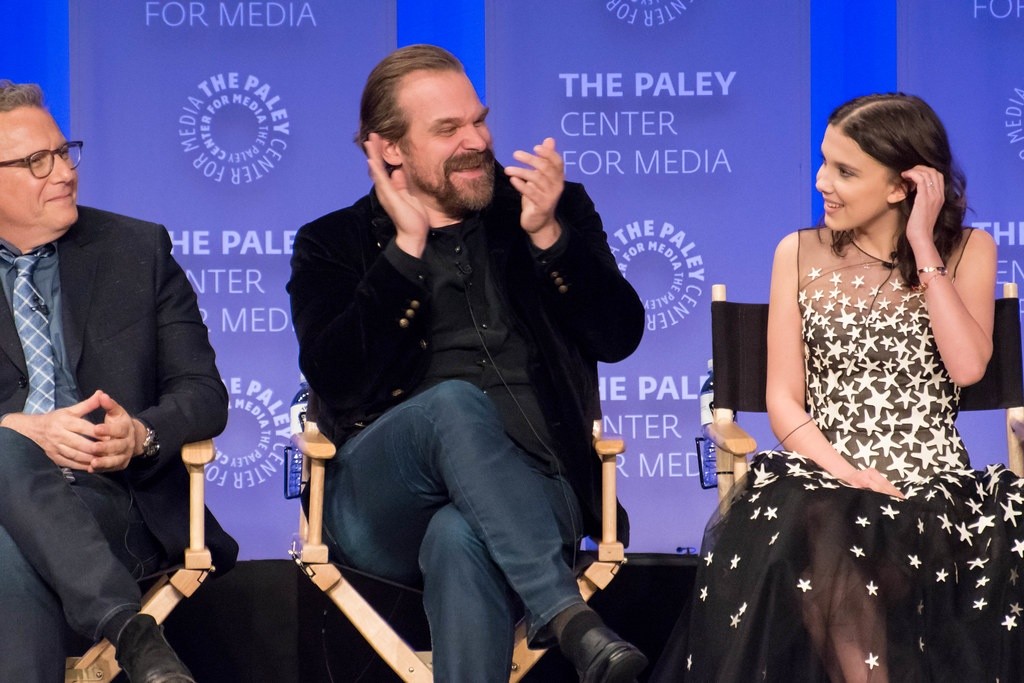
[293,420,630,681]
[705,281,1023,517]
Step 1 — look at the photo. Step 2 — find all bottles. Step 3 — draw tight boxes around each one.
[289,372,309,496]
[698,360,737,485]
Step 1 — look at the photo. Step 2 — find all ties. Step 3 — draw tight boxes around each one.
[0,248,75,484]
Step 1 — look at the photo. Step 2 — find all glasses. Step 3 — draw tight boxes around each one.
[0,140,84,178]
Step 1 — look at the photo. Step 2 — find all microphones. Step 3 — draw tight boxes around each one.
[34,296,49,314]
[455,261,473,286]
[891,252,897,269]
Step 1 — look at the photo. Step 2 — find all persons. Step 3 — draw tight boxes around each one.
[285,45,646,683]
[766,92,1024,683]
[0,85,230,683]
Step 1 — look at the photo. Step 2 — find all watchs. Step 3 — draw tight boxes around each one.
[137,418,159,457]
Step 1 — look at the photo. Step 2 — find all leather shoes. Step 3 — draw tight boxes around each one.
[115,613,195,683]
[576,626,649,683]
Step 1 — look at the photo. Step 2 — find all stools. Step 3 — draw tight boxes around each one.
[64,438,216,681]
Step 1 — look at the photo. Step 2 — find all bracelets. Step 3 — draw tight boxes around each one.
[917,266,948,290]
